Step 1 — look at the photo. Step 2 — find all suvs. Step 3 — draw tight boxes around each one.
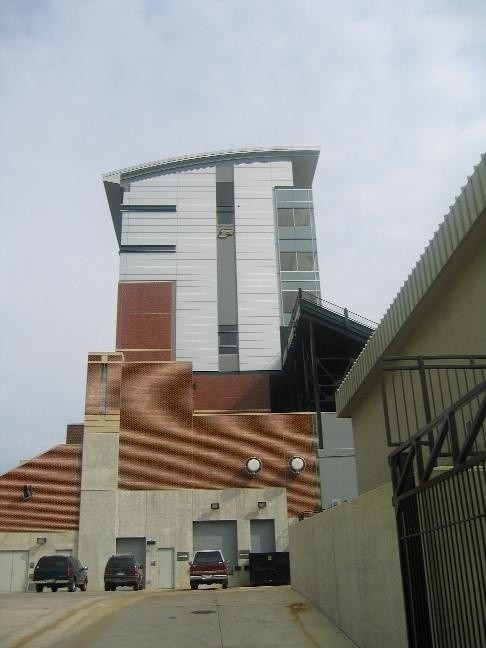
[33,554,91,592]
[102,553,145,591]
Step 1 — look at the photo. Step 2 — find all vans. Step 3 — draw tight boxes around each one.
[187,548,230,590]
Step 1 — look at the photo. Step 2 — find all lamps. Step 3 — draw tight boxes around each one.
[23,485,33,499]
[37,537,46,544]
[211,502,219,510]
[257,501,266,508]
